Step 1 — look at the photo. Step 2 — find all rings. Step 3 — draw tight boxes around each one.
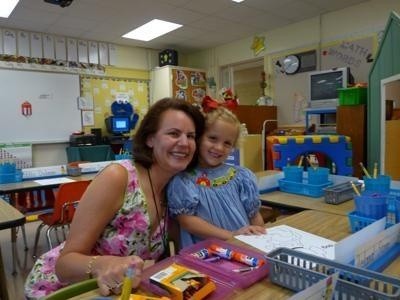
[116,282,125,289]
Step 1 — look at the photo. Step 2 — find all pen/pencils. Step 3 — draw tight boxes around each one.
[286,156,291,168]
[121,262,136,300]
[351,181,361,196]
[359,162,372,179]
[373,163,378,178]
[298,155,304,167]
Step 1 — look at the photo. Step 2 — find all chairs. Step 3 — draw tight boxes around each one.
[32,179,91,259]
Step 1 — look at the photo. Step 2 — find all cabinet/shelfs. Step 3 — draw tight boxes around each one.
[149,65,209,108]
[223,105,277,171]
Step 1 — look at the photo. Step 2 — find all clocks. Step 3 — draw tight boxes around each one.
[283,55,301,75]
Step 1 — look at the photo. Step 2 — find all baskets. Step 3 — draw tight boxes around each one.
[347,175,400,234]
[277,165,335,198]
[0,161,23,184]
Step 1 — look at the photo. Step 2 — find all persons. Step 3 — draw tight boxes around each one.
[167,97,266,250]
[23,98,207,300]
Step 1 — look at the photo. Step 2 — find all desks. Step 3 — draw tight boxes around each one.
[250,170,363,215]
[0,159,129,251]
[67,210,400,300]
[66,145,115,163]
[302,108,337,134]
[0,198,26,300]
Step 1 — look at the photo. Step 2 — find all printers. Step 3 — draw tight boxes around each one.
[70,134,96,147]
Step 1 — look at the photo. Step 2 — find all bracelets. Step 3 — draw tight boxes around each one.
[87,257,98,279]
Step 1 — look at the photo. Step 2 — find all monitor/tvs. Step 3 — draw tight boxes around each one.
[109,116,130,136]
[308,67,354,107]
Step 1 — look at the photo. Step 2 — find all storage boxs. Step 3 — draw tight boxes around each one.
[335,87,368,180]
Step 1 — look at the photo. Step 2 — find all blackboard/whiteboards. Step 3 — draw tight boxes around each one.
[0,67,83,146]
